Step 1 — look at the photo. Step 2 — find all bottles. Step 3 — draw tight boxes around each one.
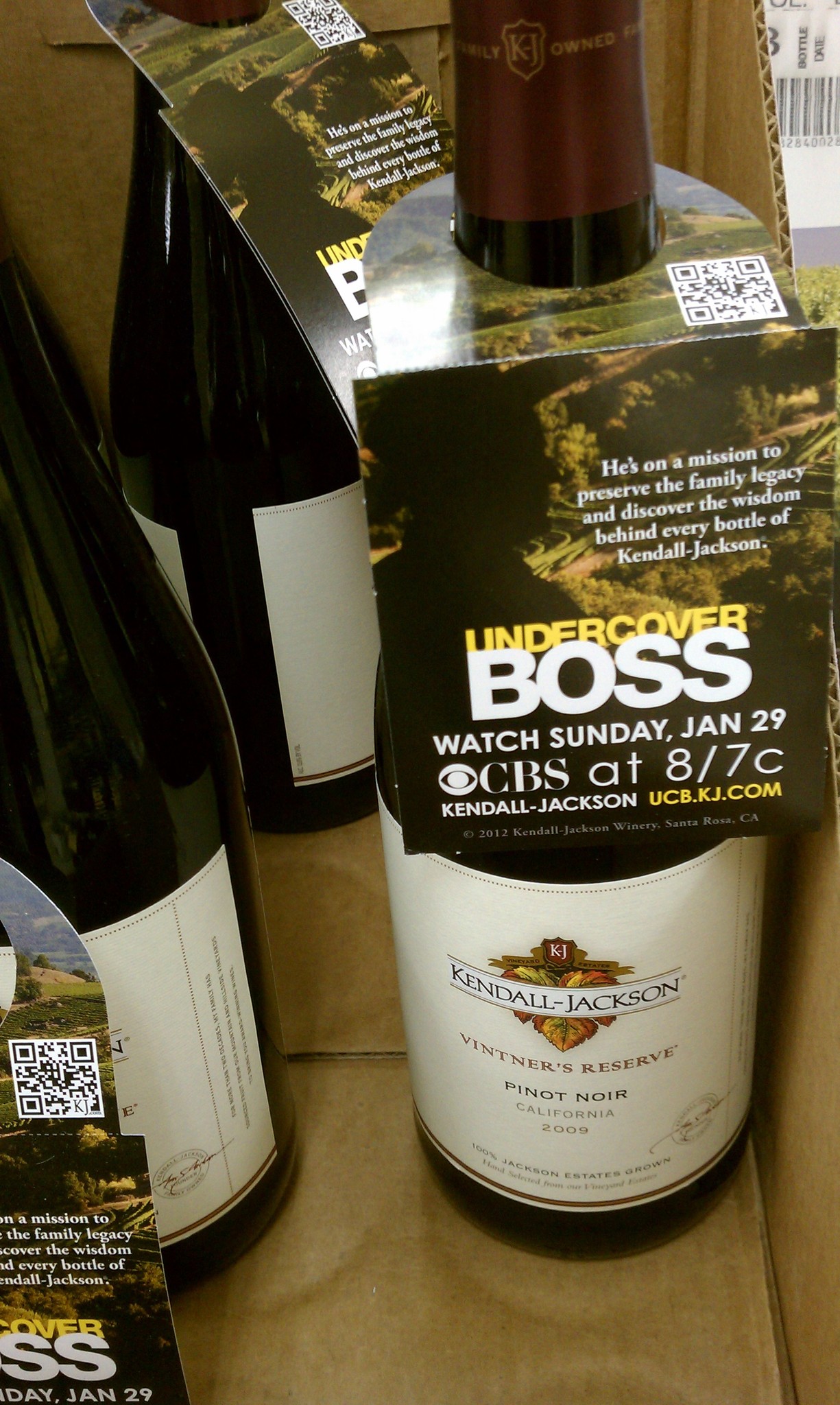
[373,1,767,1266]
[0,227,296,1305]
[106,0,378,837]
